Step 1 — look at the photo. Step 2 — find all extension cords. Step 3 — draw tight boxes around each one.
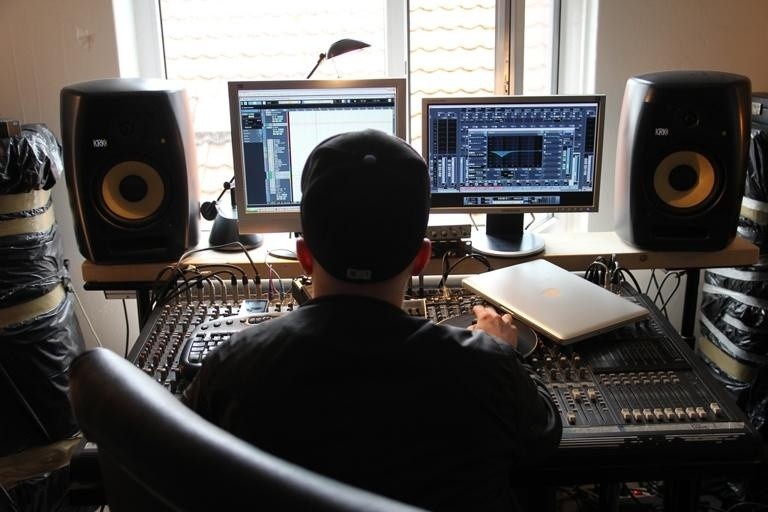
[589,485,657,506]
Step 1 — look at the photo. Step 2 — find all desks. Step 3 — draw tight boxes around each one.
[79,231,758,349]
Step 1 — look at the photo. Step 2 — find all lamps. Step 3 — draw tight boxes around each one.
[202,41,370,251]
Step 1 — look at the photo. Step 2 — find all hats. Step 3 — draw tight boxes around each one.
[299,129,430,284]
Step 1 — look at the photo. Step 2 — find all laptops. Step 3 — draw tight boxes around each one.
[462,258,650,345]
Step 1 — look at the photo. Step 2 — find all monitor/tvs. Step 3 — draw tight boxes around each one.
[421,94,606,257]
[228,79,405,260]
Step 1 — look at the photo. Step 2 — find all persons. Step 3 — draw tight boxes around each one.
[179,130,562,512]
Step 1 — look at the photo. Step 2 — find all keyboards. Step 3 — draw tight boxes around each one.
[179,312,289,371]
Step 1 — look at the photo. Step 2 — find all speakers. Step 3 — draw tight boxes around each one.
[59,77,199,265]
[614,70,752,253]
